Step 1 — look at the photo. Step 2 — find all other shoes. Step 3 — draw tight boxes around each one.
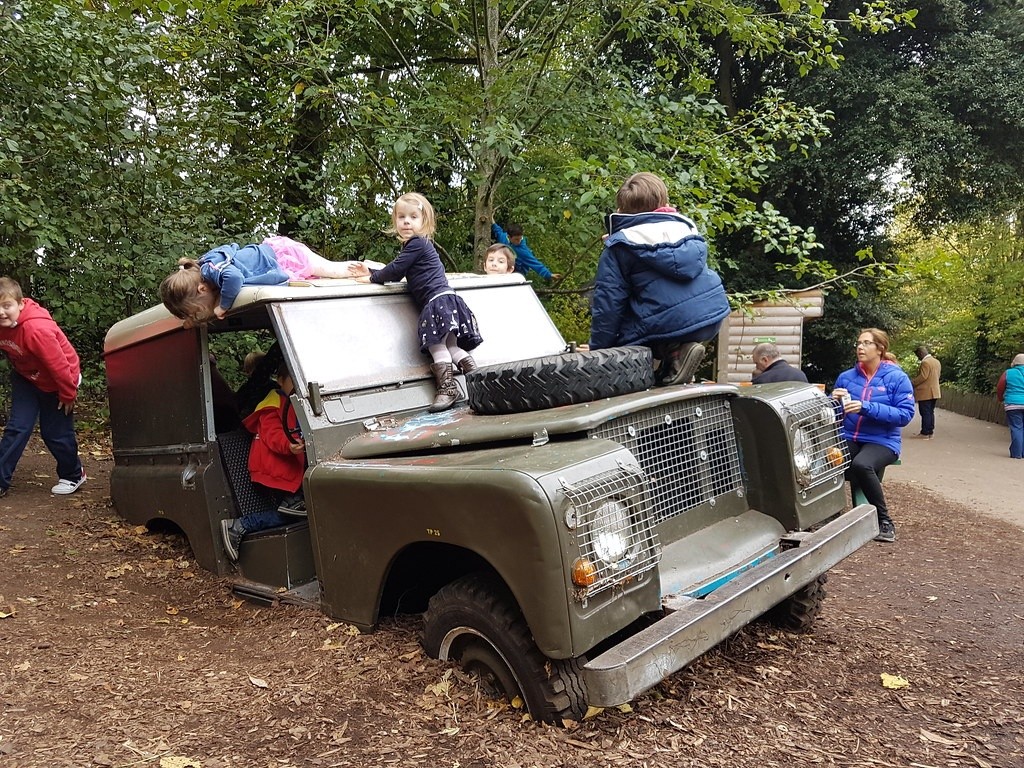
[277,497,308,516]
[660,341,706,386]
[221,519,247,561]
[913,434,929,440]
[929,434,934,440]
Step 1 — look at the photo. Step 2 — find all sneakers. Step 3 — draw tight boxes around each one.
[874,523,896,542]
[51,467,87,495]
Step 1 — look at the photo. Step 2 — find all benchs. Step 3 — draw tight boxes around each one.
[855,460,902,506]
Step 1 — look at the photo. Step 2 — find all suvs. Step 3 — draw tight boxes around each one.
[100,265,881,726]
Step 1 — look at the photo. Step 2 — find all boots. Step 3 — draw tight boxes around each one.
[458,355,477,375]
[429,361,461,412]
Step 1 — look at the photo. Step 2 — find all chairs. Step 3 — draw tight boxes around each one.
[218,427,310,537]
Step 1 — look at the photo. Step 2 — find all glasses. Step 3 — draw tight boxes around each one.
[855,340,875,346]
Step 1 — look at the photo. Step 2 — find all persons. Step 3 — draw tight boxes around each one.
[492,211,561,280]
[0,277,87,497]
[347,192,484,412]
[220,361,308,561]
[911,346,941,440]
[828,328,915,543]
[210,351,280,435]
[751,342,808,384]
[589,172,730,386]
[483,242,517,274]
[997,353,1024,459]
[158,235,358,329]
